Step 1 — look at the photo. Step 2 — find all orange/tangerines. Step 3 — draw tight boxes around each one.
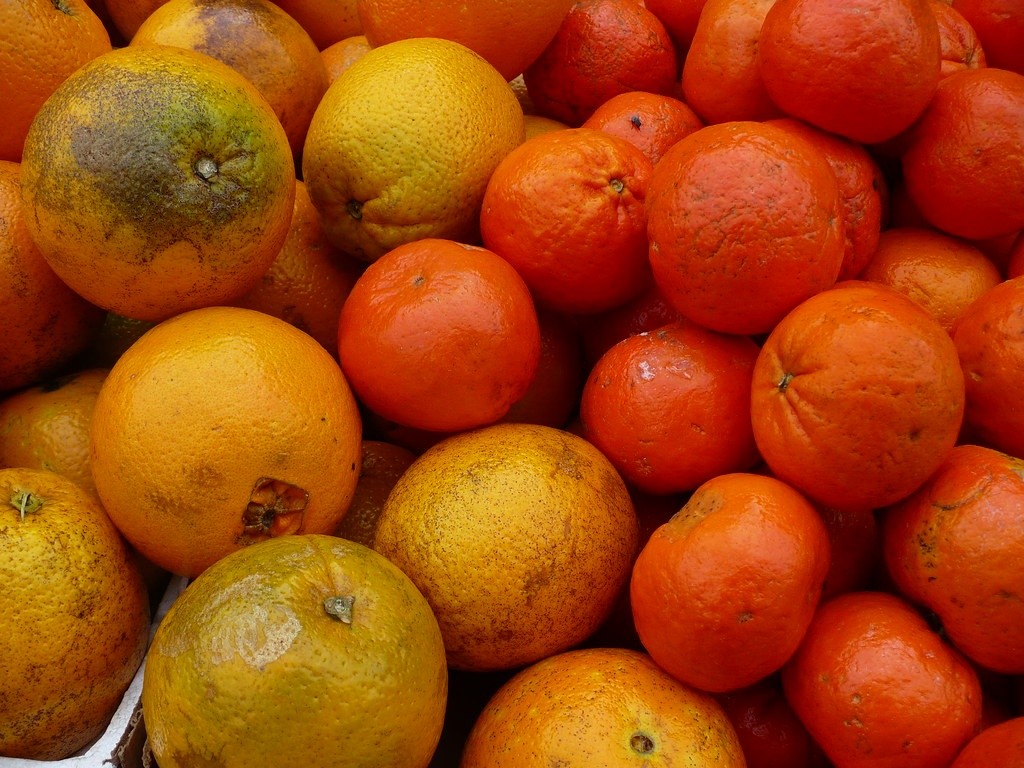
[0,0,1024,768]
